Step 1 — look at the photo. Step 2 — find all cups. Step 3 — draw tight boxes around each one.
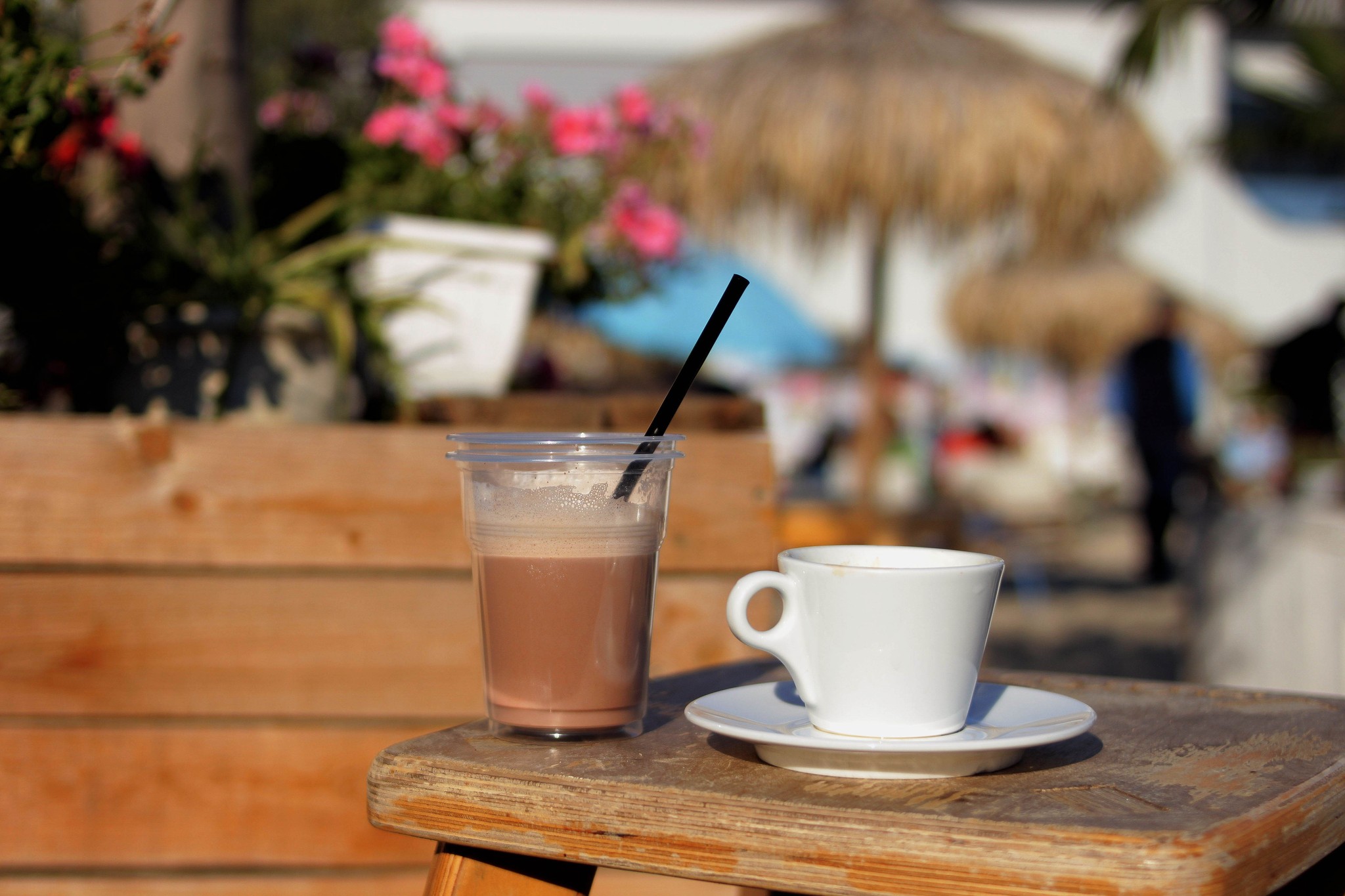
[447,428,683,742]
[725,544,1006,735]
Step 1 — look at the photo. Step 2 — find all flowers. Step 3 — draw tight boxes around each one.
[259,20,695,282]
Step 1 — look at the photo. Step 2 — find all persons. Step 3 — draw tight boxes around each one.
[1131,300,1192,582]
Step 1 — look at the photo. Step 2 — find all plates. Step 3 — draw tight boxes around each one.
[684,677,1098,780]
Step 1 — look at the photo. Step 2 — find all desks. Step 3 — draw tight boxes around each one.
[364,660,1345,896]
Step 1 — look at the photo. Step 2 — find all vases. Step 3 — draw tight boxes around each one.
[350,212,552,399]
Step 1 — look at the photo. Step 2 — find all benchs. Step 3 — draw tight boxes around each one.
[0,416,784,896]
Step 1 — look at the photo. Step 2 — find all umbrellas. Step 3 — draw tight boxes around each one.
[612,0,1169,456]
[952,238,1260,462]
